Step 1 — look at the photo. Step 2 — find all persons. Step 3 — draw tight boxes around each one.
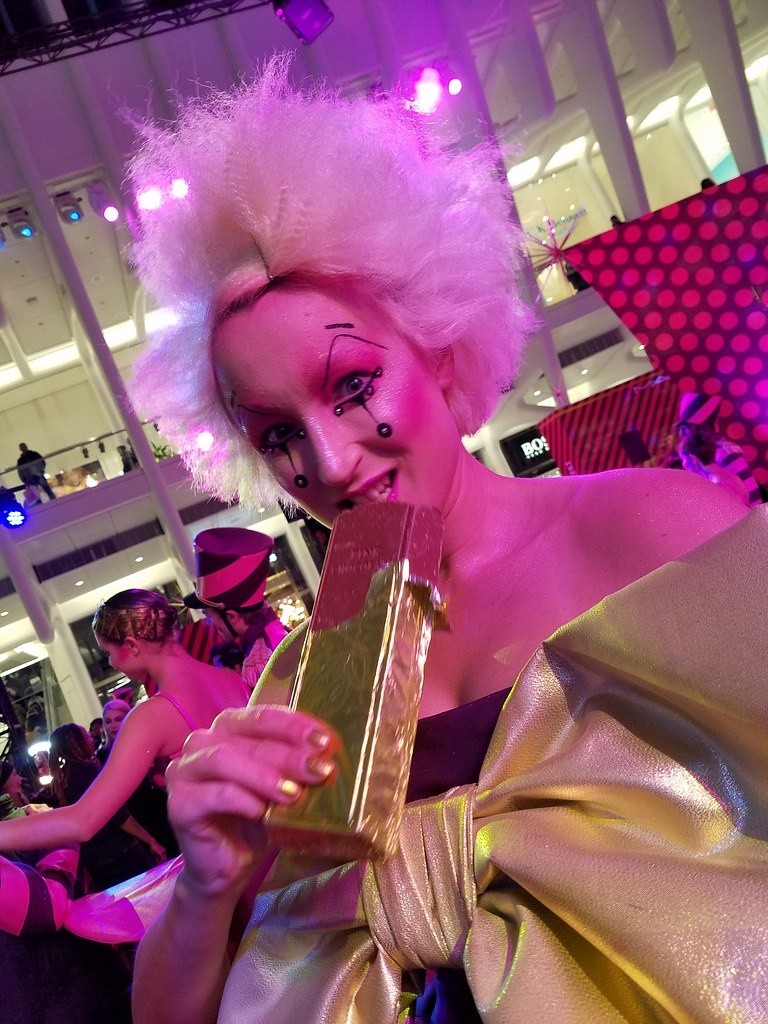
[131,44,768,1024]
[664,391,762,510]
[17,443,56,505]
[0,527,289,1024]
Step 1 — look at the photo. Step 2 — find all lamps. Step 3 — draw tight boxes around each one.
[53,192,84,224]
[7,208,36,239]
[87,178,119,223]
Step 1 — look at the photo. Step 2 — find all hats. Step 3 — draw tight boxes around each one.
[183,528,274,611]
[674,392,722,427]
